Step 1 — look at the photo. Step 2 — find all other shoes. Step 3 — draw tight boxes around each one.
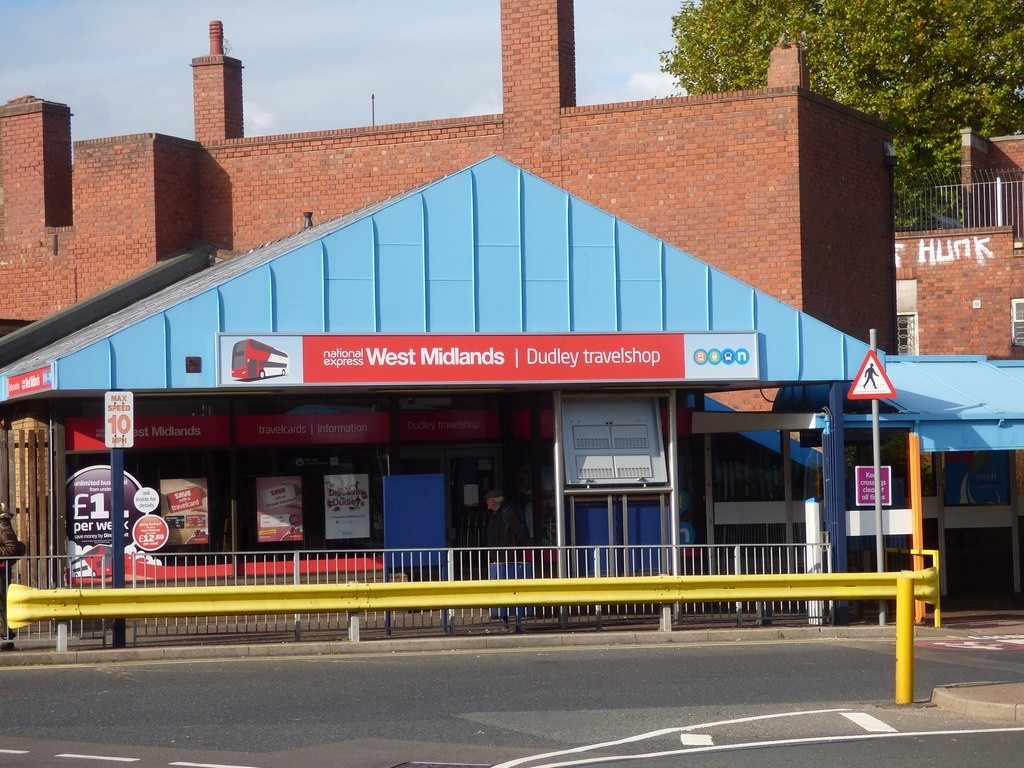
[4,633,12,640]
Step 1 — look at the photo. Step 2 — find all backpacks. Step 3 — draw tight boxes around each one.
[502,503,530,540]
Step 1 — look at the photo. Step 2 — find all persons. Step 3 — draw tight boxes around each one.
[845,498,869,624]
[482,489,530,620]
[0,502,18,651]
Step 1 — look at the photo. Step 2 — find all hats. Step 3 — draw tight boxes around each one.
[482,489,503,499]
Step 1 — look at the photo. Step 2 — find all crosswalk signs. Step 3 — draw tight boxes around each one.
[846,349,898,399]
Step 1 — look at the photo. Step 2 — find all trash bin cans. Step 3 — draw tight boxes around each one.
[489,563,534,618]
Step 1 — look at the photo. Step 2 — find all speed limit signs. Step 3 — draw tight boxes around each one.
[105,392,134,449]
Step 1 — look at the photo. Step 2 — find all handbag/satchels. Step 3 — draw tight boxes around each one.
[8,541,25,565]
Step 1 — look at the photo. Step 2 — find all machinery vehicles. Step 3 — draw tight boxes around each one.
[232,338,289,381]
